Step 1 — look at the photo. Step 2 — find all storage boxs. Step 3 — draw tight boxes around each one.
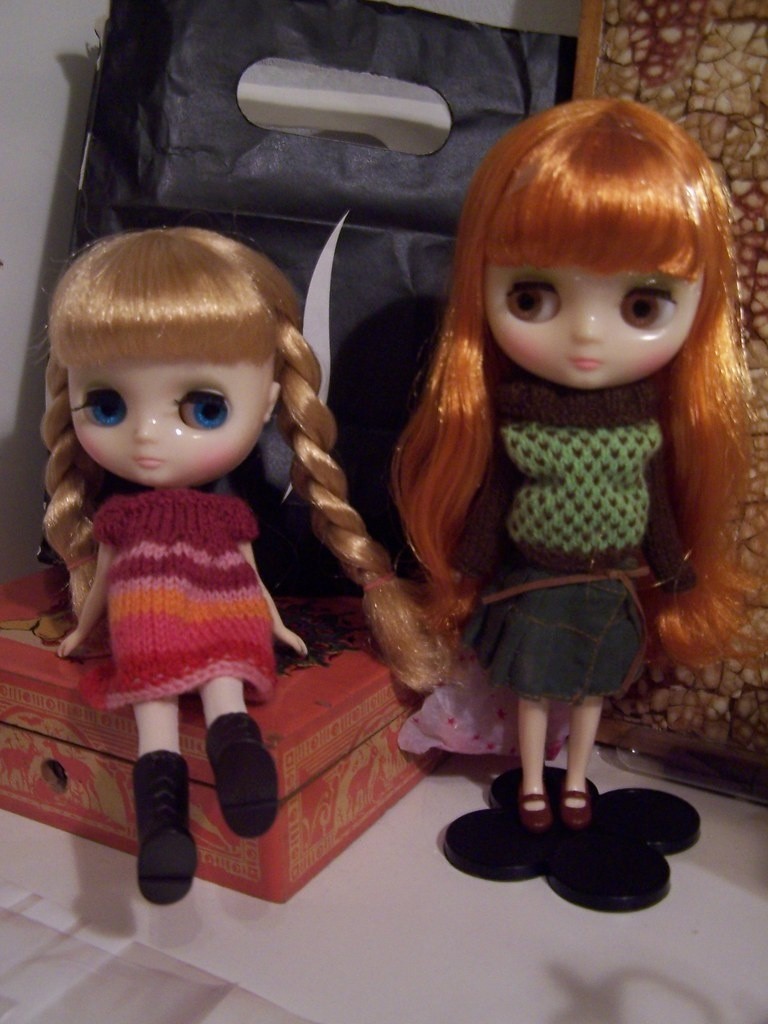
[0,572,446,902]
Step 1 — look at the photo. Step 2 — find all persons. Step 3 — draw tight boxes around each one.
[39,227,455,907]
[388,96,750,832]
